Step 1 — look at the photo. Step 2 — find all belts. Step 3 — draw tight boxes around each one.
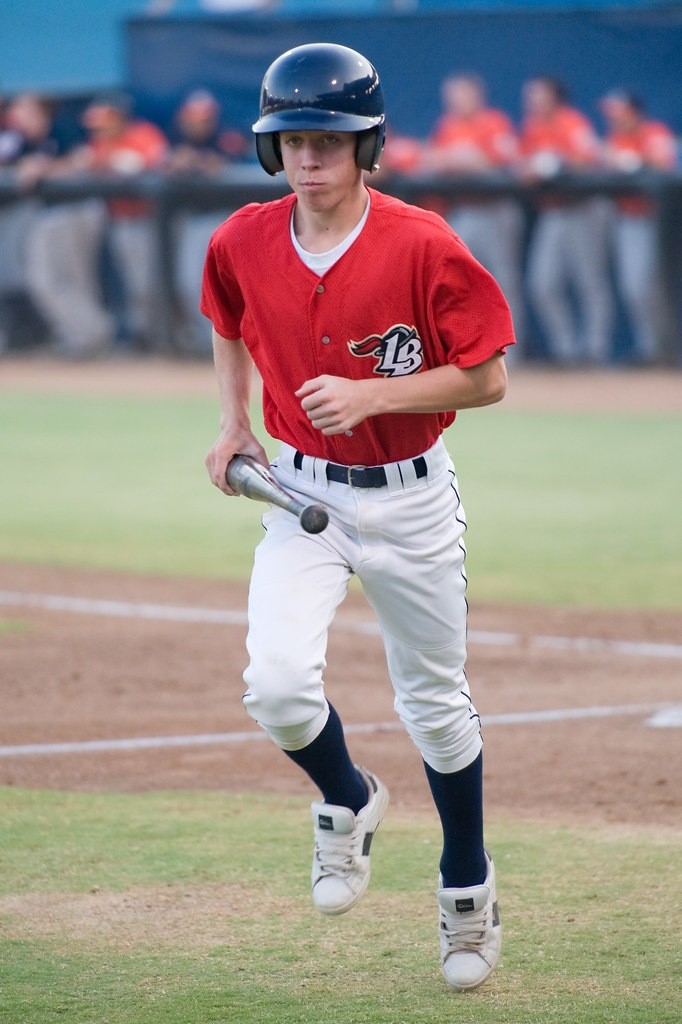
[294,451,427,491]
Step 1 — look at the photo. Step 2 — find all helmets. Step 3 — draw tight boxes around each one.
[252,43,387,177]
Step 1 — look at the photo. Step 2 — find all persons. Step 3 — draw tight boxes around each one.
[0,72,681,362]
[205,44,516,989]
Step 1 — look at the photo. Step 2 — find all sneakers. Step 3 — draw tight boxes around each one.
[311,764,390,916]
[437,851,503,990]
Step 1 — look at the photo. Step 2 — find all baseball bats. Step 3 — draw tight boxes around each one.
[226,455,329,534]
